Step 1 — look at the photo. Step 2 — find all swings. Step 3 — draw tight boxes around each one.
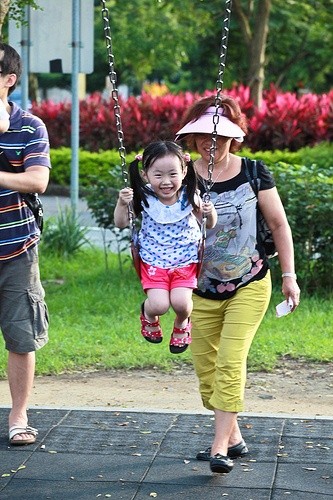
[100,0,233,279]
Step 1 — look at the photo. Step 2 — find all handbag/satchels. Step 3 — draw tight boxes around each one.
[244,157,275,255]
[26,193,45,233]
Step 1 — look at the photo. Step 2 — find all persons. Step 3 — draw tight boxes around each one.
[114,141,218,354]
[176,96,301,472]
[26,71,170,105]
[0,43,51,442]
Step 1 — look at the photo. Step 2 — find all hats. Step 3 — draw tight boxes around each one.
[175,105,247,138]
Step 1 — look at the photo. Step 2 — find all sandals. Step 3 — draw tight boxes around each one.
[196,441,250,460]
[209,453,235,473]
[168,315,192,353]
[140,302,163,344]
[7,422,37,445]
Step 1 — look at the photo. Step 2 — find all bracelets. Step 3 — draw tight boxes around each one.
[280,273,298,280]
